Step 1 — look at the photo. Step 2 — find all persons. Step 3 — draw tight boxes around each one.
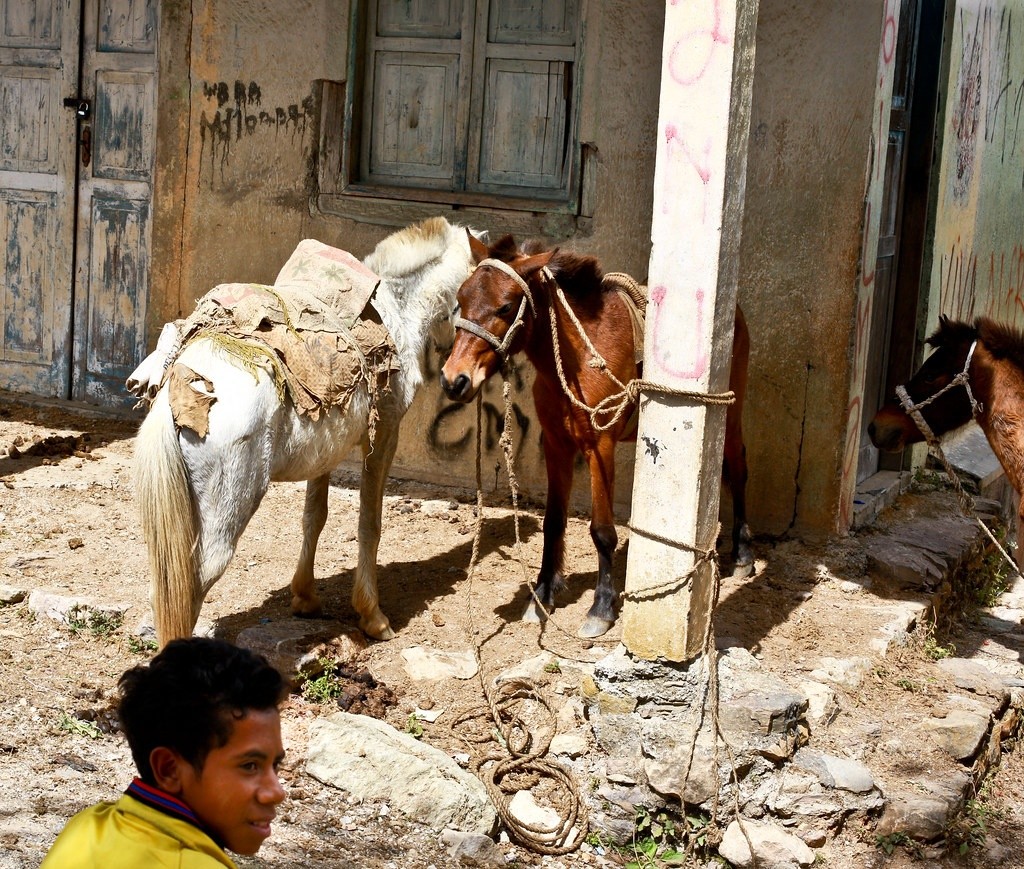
[40,637,288,869]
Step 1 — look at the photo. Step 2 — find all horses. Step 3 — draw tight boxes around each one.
[866,312,1024,531]
[136,216,749,653]
